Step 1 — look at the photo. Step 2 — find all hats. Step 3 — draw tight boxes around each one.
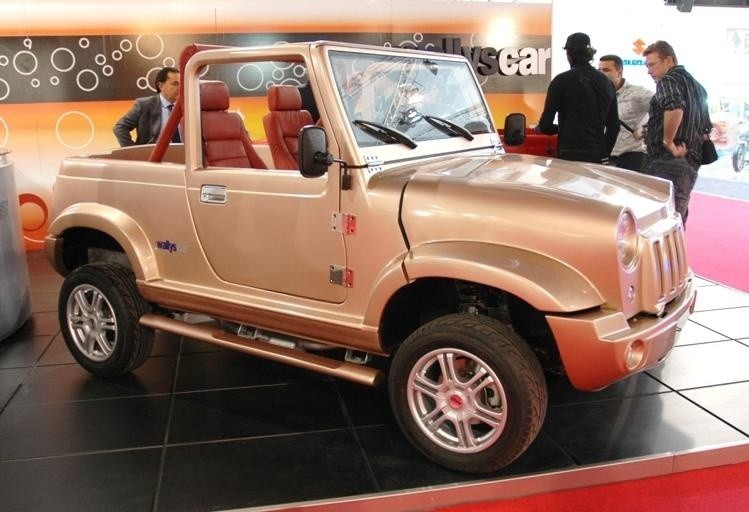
[562,33,590,49]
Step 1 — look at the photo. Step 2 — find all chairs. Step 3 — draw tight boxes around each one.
[177,80,268,170]
[263,84,318,171]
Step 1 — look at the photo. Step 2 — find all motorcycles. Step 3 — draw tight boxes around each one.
[730,112,749,173]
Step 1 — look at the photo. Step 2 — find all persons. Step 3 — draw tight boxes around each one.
[538,33,620,165]
[643,41,713,232]
[113,67,184,147]
[598,56,655,174]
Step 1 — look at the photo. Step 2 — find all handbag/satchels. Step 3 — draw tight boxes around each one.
[702,141,718,164]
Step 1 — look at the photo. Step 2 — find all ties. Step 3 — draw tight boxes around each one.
[167,104,182,142]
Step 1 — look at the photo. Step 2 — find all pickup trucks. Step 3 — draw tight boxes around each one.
[41,35,705,469]
